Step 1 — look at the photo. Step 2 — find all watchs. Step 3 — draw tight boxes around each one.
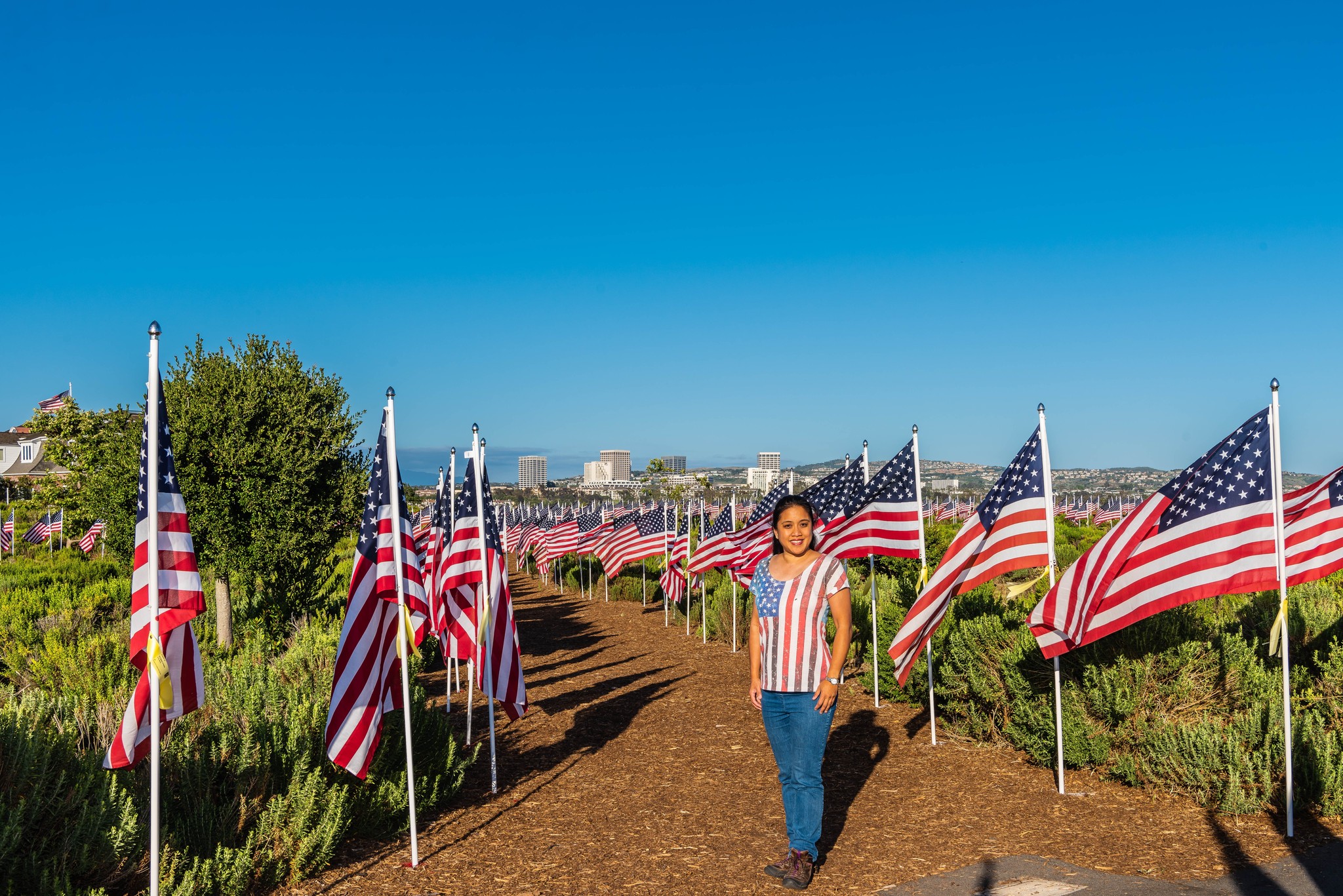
[824,676,841,686]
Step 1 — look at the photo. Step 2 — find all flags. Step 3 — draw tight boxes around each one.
[922,495,981,526]
[1024,401,1281,659]
[78,515,107,553]
[323,403,434,784]
[1283,465,1343,586]
[38,389,70,414]
[4,509,13,542]
[887,425,1053,686]
[1,514,10,551]
[20,511,51,545]
[1053,495,1143,527]
[490,454,873,608]
[101,357,208,775]
[50,510,63,532]
[412,441,530,721]
[808,434,923,560]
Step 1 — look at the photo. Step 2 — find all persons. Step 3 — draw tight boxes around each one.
[747,495,852,889]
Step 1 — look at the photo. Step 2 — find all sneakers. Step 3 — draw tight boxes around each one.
[764,852,792,878]
[783,847,813,890]
